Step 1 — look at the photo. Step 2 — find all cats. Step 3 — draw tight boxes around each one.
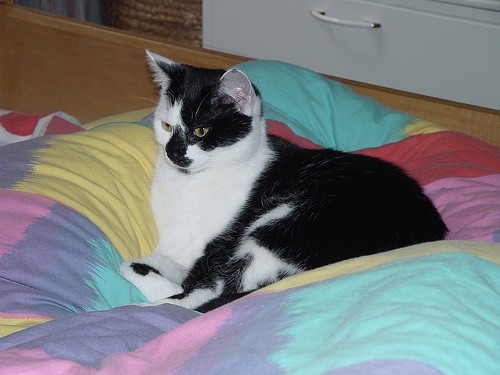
[119,47,449,316]
